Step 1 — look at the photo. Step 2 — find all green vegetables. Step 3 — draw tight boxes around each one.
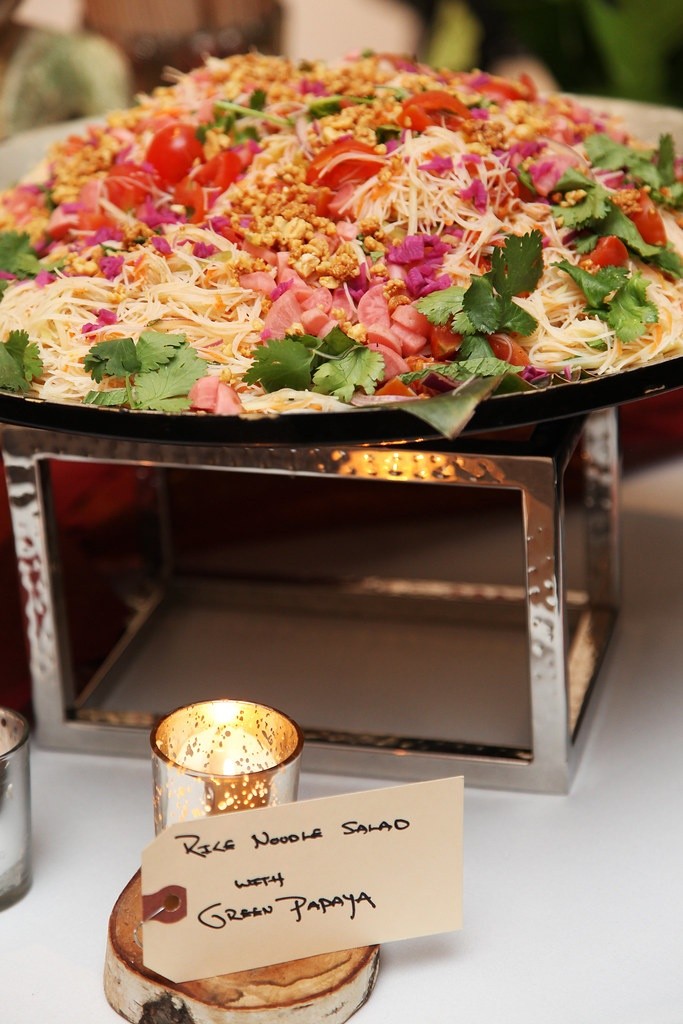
[0,87,683,412]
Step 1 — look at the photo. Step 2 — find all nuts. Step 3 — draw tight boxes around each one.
[0,53,672,349]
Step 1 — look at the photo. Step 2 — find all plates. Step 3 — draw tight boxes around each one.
[0,85,683,441]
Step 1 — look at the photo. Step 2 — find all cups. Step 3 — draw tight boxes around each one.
[149,698,304,838]
[0,708,33,913]
